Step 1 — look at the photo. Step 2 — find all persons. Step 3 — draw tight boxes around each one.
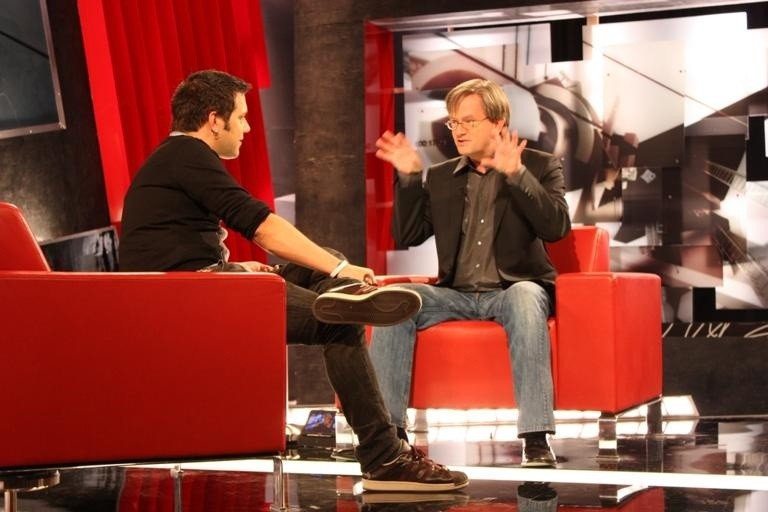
[330,76,572,469]
[119,71,472,494]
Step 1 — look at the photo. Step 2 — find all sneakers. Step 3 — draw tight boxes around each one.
[311,282,425,328]
[521,433,557,468]
[360,494,470,512]
[361,438,470,491]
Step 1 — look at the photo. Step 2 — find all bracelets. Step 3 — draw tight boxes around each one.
[330,259,348,277]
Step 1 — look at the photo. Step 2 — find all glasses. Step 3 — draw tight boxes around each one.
[444,116,489,130]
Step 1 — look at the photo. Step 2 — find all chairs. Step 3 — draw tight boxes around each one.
[1,198,291,512]
[332,225,667,465]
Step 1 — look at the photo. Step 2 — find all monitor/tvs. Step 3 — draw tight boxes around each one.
[362,0,768,339]
